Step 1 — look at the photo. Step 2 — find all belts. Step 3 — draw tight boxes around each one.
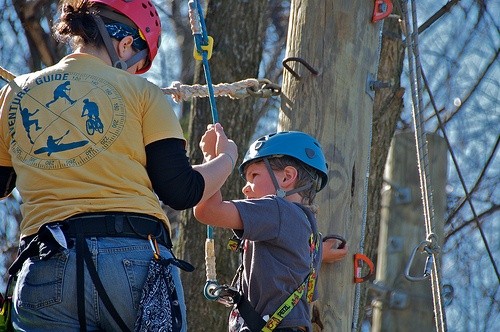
[67,215,172,247]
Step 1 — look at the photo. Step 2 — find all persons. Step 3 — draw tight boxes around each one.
[193,122,348,332]
[0,0,239,332]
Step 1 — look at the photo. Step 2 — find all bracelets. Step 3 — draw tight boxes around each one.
[223,152,234,171]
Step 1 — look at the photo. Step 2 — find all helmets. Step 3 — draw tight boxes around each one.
[80,1,162,74]
[238,130,328,198]
